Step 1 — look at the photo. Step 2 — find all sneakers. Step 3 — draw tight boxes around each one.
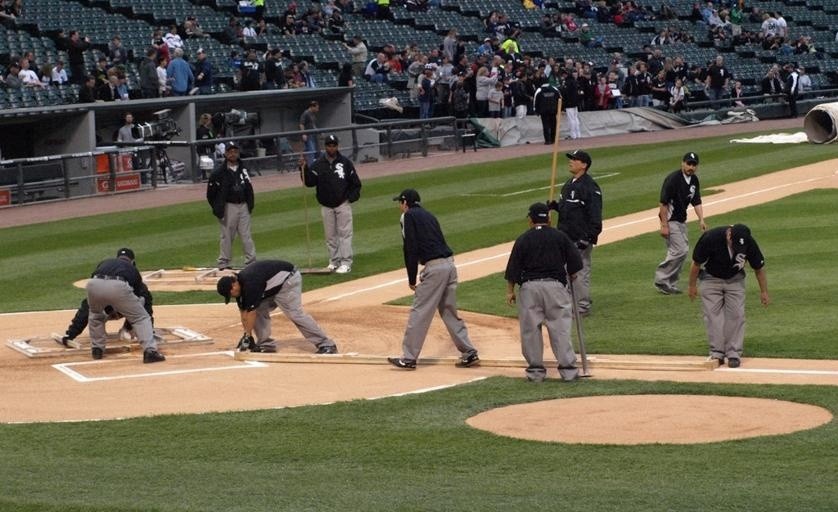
[118,328,136,342]
[455,354,479,367]
[327,264,337,270]
[92,347,103,359]
[315,345,337,354]
[144,351,165,363]
[728,358,740,368]
[654,283,682,295]
[388,358,417,368]
[251,346,276,352]
[335,265,351,274]
[719,358,724,365]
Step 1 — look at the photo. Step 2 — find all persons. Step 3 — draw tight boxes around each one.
[544,149,602,319]
[87,248,166,364]
[386,189,480,371]
[296,134,362,275]
[688,224,770,368]
[217,260,338,355]
[63,279,155,346]
[0,1,838,182]
[655,152,708,295]
[503,202,583,383]
[206,143,256,271]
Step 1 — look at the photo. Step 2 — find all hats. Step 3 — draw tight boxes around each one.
[566,150,591,165]
[226,141,238,150]
[217,277,233,304]
[117,248,135,266]
[325,135,338,145]
[527,203,549,218]
[731,224,753,253]
[393,190,420,204]
[683,152,698,164]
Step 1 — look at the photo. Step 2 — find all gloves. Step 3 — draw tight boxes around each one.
[577,240,589,250]
[546,200,556,211]
[238,338,250,351]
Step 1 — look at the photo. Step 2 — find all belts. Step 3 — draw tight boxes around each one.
[287,265,298,279]
[98,276,126,282]
[530,278,557,281]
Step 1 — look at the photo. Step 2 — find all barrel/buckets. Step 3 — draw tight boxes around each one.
[96,146,117,174]
[515,104,528,118]
